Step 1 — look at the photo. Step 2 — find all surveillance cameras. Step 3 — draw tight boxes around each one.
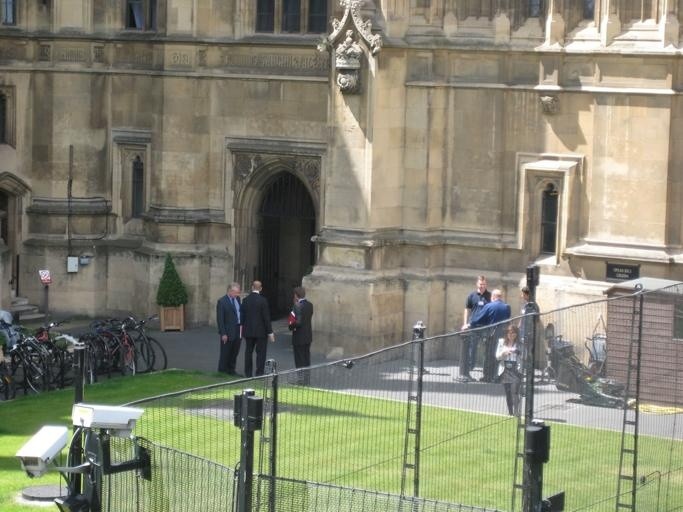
[72,403,144,430]
[14,425,68,471]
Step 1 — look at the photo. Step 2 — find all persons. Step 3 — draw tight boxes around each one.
[518,287,541,374]
[464,288,511,381]
[215,282,243,377]
[239,280,275,380]
[468,275,492,371]
[288,287,313,384]
[495,324,522,418]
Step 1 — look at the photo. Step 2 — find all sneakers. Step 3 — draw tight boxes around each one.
[467,362,500,384]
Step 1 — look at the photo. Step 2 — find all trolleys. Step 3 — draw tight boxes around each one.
[543,314,624,409]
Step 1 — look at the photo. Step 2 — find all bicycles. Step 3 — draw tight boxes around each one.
[0,313,167,401]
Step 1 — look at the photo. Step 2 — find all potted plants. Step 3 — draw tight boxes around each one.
[157,251,188,332]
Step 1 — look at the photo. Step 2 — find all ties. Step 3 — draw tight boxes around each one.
[231,297,235,305]
